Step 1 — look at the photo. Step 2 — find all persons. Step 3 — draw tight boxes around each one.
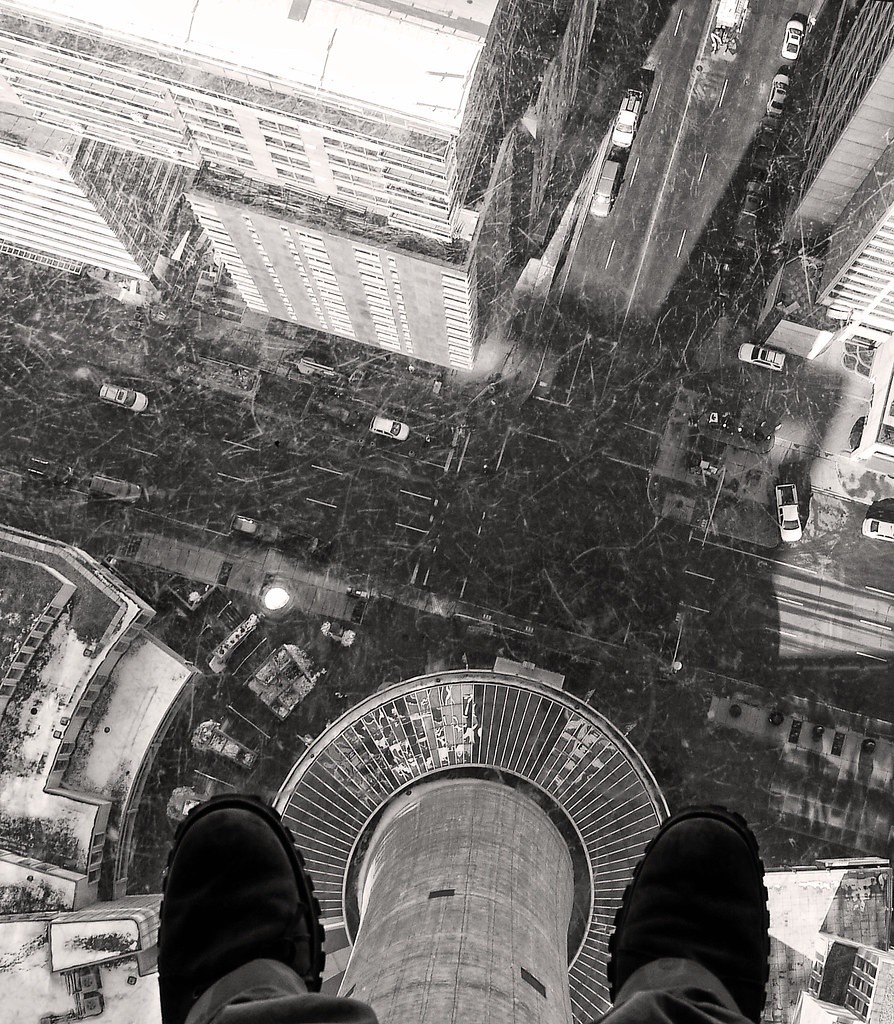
[154,792,775,1024]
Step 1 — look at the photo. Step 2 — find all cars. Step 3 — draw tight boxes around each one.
[65,18,819,828]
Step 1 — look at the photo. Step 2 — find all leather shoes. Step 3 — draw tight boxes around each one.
[151,792,327,1024]
[606,803,771,1024]
